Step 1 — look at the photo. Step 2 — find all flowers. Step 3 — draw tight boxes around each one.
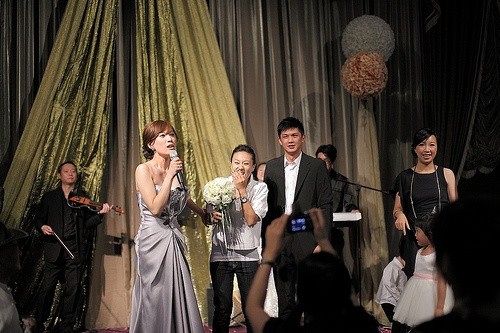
[203,175,237,250]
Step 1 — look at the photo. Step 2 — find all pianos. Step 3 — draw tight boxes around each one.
[332,211,365,305]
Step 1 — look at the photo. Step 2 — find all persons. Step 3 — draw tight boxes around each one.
[1,221,45,333]
[30,162,114,333]
[129,121,211,333]
[262,250,380,333]
[375,255,407,324]
[260,117,333,323]
[232,162,279,318]
[392,129,456,279]
[392,212,454,327]
[316,144,359,295]
[203,144,269,333]
[409,195,500,333]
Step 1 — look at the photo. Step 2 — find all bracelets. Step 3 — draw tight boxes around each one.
[394,209,403,220]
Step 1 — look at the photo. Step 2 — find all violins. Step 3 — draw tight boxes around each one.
[67,187,128,216]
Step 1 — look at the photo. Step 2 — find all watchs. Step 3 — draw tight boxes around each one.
[240,197,249,203]
[258,259,274,267]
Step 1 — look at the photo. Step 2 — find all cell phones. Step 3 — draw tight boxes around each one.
[286,213,311,234]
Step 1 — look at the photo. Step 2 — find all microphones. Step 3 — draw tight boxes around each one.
[168,150,185,189]
